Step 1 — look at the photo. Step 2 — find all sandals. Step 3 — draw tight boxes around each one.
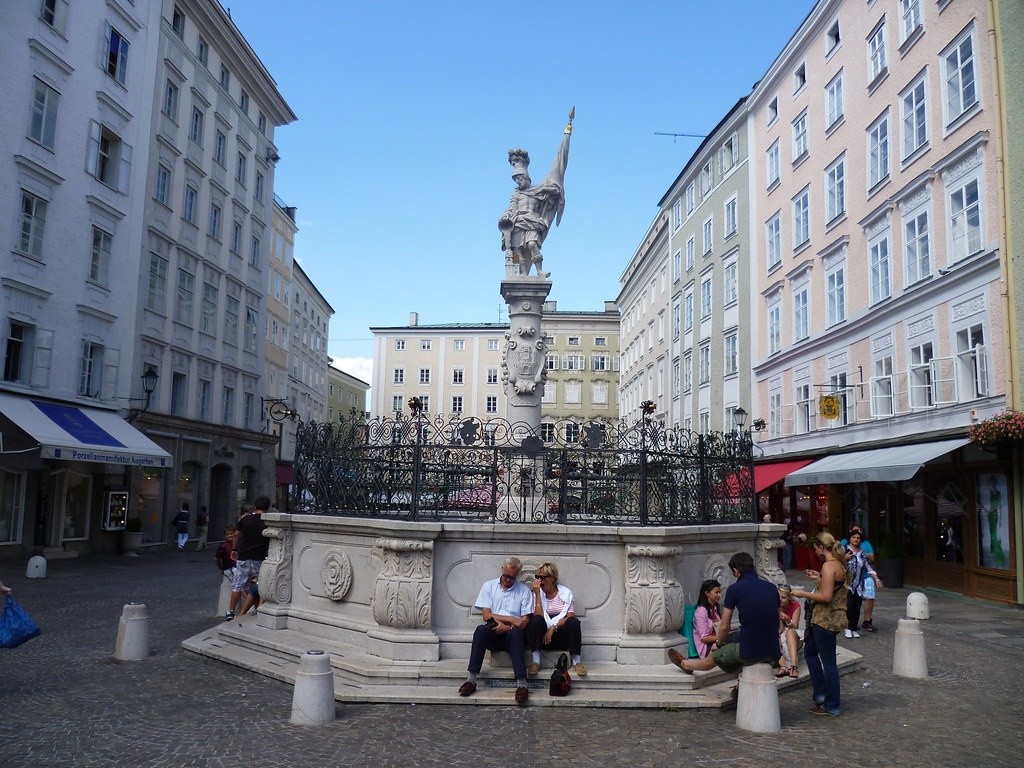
[789,665,798,678]
[775,666,790,677]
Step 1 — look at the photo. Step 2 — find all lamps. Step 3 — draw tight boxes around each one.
[135,366,159,415]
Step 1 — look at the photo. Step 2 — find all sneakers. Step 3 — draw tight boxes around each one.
[225,610,234,621]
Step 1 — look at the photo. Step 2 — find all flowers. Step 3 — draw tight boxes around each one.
[967,409,1024,447]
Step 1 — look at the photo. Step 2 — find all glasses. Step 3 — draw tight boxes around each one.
[778,584,791,592]
[534,575,550,580]
[503,575,516,581]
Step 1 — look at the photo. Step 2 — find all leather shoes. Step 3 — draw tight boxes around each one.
[458,681,477,696]
[515,686,529,701]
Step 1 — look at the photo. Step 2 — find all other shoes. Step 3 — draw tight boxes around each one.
[247,605,255,614]
[195,549,201,552]
[573,663,587,675]
[204,546,208,550]
[668,648,693,674]
[862,619,874,631]
[528,662,539,674]
[852,631,860,638]
[809,704,839,717]
[176,548,179,552]
[178,545,183,552]
[844,629,852,638]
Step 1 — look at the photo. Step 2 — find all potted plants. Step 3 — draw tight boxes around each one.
[123,517,144,552]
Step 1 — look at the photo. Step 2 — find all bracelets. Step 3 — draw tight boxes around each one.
[786,620,792,626]
[554,624,558,632]
[232,549,237,551]
[509,625,512,630]
[536,603,542,606]
[876,579,880,582]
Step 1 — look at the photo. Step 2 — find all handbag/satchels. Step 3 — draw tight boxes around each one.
[550,653,571,696]
[0,595,42,648]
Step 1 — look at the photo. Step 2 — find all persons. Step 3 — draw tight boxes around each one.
[789,532,851,717]
[498,149,558,277]
[0,581,12,596]
[667,553,782,698]
[982,474,1006,569]
[781,519,884,638]
[195,506,210,552]
[691,579,804,681]
[214,497,272,622]
[458,557,535,702]
[527,562,587,676]
[176,503,190,552]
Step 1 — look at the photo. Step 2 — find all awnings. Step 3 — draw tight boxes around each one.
[784,437,973,488]
[0,389,174,467]
[693,459,816,503]
